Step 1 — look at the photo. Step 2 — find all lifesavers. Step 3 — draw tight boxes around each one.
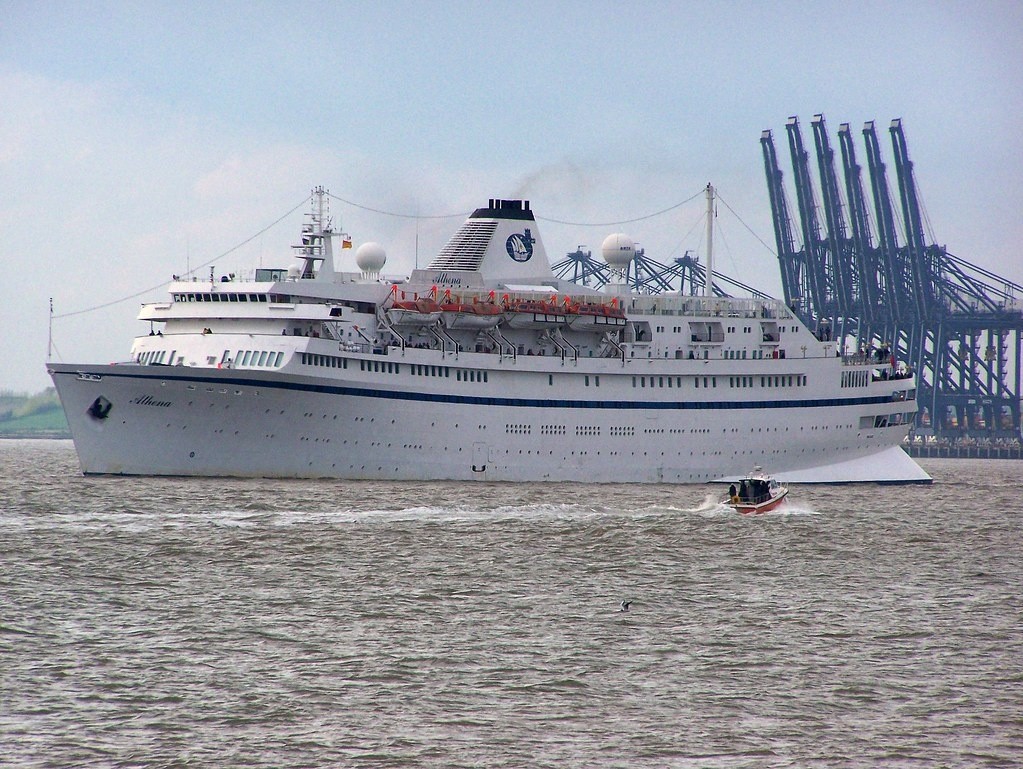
[732,496,740,504]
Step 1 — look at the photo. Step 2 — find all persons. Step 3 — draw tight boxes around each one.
[715,303,720,315]
[150,330,162,335]
[282,329,286,335]
[790,304,796,313]
[729,481,773,504]
[527,348,533,355]
[637,329,646,341]
[305,326,315,337]
[202,327,213,334]
[854,346,913,380]
[537,348,544,355]
[814,326,833,341]
[386,340,430,350]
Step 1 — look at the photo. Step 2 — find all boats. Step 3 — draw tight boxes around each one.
[720,475,789,515]
[386,298,443,325]
[439,302,502,329]
[501,298,565,330]
[563,303,626,334]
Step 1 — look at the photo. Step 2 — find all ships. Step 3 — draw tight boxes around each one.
[43,178,931,488]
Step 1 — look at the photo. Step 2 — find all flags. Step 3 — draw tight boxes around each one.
[341,241,352,248]
